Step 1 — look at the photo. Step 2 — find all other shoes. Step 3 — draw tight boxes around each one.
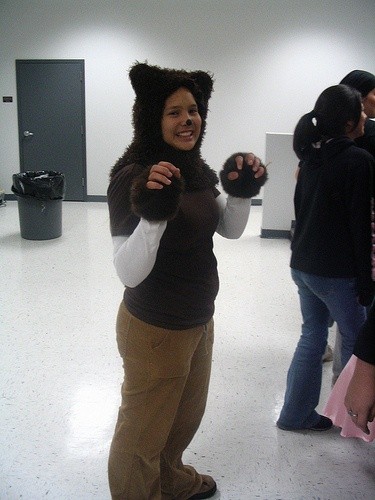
[277,415,332,432]
[321,344,334,362]
[187,474,216,500]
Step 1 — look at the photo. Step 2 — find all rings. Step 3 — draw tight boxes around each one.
[348,411,357,417]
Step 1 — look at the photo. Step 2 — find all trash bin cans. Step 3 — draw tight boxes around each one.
[12,170,66,241]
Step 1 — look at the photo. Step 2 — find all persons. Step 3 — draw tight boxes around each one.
[276,70,375,443]
[106,64,268,500]
